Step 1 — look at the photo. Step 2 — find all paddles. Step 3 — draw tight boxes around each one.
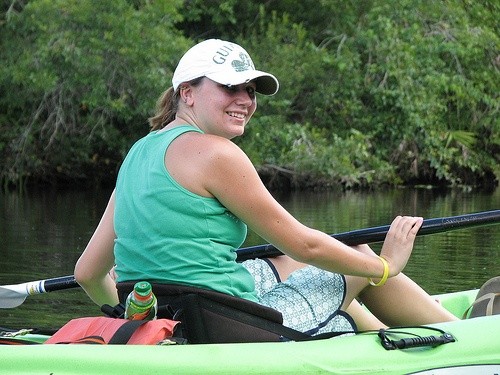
[0,209,500,308]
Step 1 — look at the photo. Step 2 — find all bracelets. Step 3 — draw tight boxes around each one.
[369,255,389,288]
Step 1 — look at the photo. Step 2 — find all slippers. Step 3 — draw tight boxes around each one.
[462,275,500,319]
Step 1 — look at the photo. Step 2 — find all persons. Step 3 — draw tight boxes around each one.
[73,38,459,343]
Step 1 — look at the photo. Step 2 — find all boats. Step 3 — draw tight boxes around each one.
[0,286,499,375]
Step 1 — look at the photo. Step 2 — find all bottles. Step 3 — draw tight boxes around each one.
[123,282,158,320]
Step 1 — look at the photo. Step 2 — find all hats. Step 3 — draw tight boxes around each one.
[171,38,280,96]
